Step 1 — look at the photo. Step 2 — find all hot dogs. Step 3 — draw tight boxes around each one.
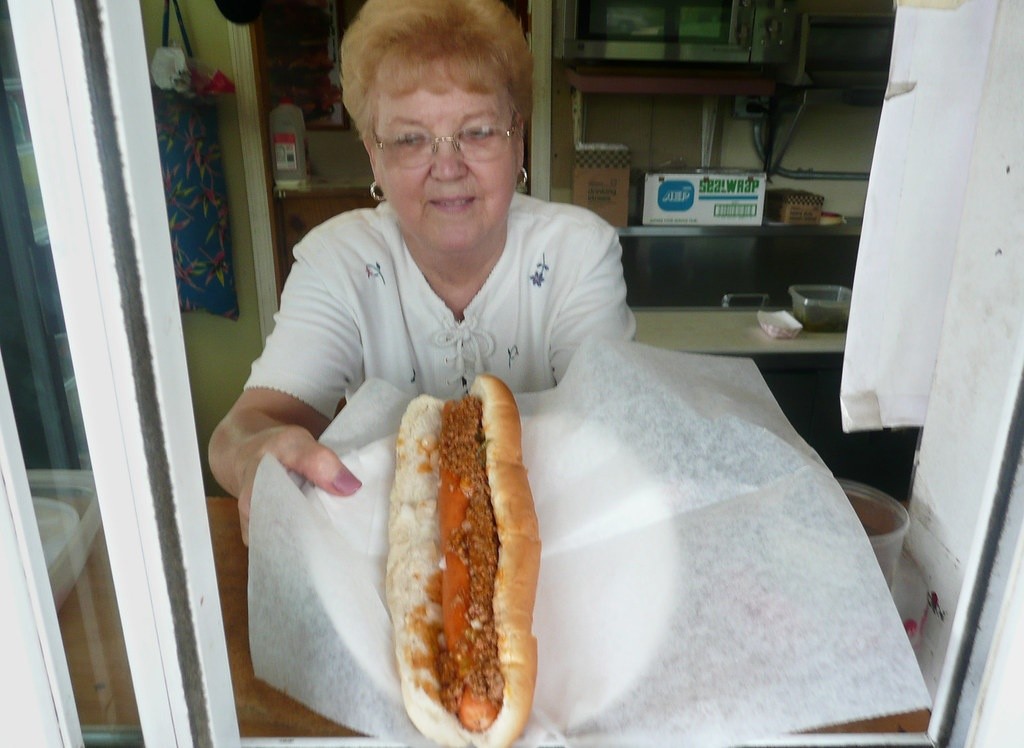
[385,373,541,748]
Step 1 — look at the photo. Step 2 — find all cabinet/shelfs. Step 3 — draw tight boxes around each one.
[227,0,895,370]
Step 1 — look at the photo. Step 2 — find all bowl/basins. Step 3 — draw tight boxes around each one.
[790,284,852,331]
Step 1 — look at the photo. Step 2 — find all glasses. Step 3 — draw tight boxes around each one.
[372,112,517,170]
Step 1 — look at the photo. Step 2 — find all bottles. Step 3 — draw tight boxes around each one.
[267,96,310,191]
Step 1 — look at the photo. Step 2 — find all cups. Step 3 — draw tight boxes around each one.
[832,478,910,597]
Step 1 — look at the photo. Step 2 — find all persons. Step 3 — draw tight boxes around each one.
[208,0,636,549]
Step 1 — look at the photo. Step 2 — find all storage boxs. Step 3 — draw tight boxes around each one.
[763,188,825,224]
[570,148,630,228]
[643,174,768,226]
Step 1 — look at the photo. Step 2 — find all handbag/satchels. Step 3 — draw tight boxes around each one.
[151,0,240,321]
